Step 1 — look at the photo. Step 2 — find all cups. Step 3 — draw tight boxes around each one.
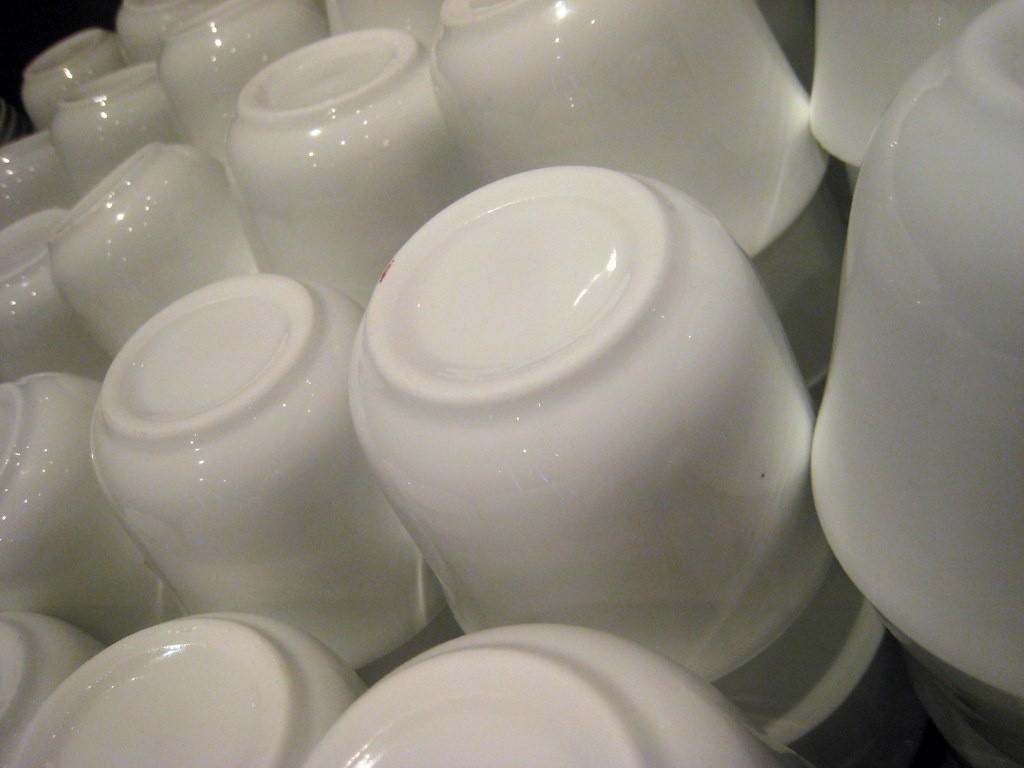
[809,0,1024,697]
[879,614,1024,768]
[1,0,915,768]
[806,0,999,166]
